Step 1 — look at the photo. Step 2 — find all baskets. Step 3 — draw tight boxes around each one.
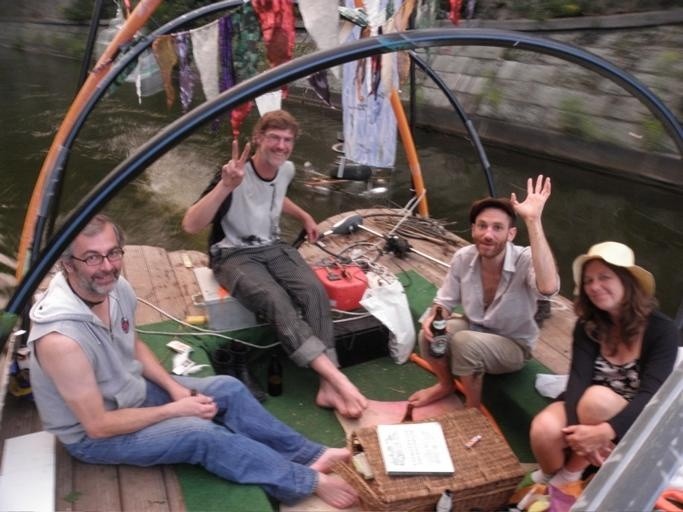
[352,408,524,512]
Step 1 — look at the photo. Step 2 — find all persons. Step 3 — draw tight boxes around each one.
[189,510,191,510]
[407,172,561,409]
[27,213,362,509]
[528,241,678,489]
[182,110,369,420]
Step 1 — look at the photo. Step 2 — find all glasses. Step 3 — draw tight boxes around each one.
[71,251,124,265]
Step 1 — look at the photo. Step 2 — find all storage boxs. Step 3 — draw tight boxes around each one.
[329,407,528,512]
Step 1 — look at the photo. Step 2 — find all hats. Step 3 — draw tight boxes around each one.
[572,241,656,297]
[470,198,516,221]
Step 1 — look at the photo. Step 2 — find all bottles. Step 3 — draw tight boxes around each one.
[428,305,449,360]
[436,488,454,512]
